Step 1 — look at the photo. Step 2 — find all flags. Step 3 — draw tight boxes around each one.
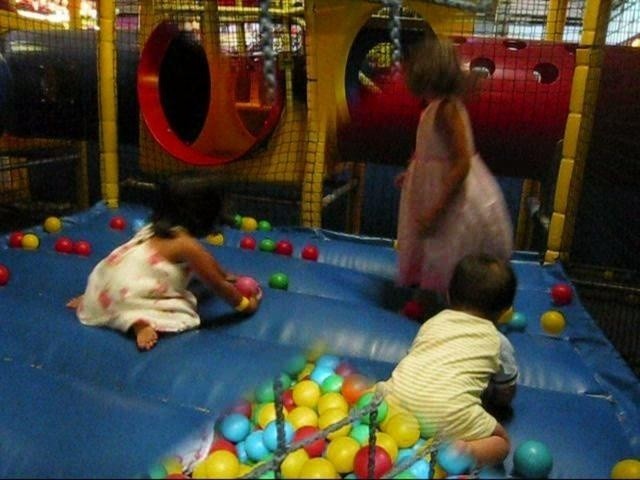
[1,203,321,294]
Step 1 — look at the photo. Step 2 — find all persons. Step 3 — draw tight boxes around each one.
[382,250,523,469]
[389,28,517,314]
[65,170,258,352]
